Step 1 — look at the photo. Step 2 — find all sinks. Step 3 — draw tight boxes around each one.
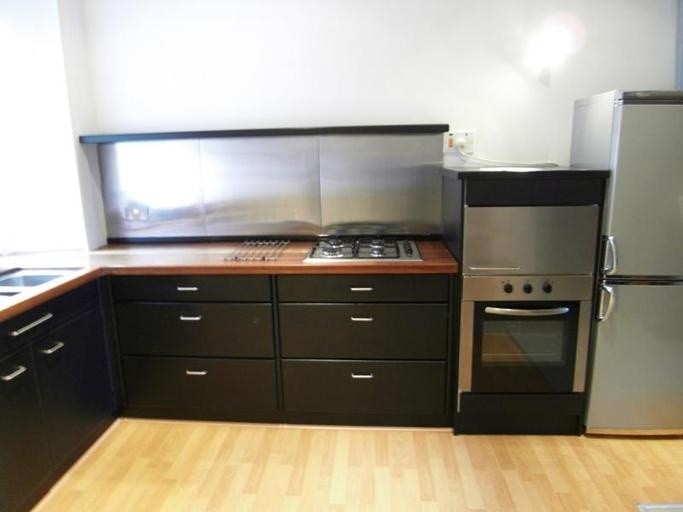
[0,268,85,287]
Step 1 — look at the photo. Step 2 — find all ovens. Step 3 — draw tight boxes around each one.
[457,273,590,395]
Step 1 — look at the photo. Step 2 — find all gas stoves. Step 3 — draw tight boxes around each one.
[300,235,422,263]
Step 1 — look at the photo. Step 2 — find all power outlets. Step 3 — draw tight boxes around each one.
[443,132,474,153]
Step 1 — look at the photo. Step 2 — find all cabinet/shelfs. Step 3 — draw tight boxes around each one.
[0,277,119,512]
[275,272,454,425]
[109,273,280,423]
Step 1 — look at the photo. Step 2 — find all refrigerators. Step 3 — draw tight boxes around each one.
[566,86,682,438]
